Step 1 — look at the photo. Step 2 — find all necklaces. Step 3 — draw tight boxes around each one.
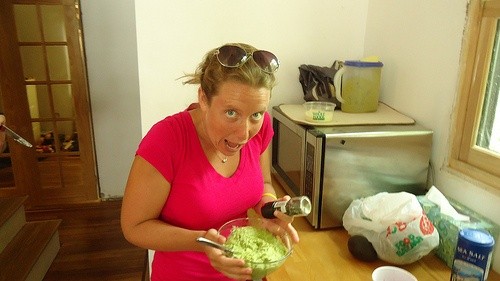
[199,107,231,163]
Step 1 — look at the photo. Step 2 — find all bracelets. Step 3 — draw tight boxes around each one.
[262,193,276,200]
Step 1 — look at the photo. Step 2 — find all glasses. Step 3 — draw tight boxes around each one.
[204,45,280,74]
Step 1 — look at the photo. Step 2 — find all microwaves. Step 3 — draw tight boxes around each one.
[269,105,434,230]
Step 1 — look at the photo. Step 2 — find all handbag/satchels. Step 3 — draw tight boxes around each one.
[342,191,439,265]
[299,60,342,110]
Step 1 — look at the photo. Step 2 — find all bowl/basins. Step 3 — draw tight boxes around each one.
[371,266,418,281]
[302,102,336,123]
[217,218,294,279]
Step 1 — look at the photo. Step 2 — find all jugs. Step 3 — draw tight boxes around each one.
[333,59,383,114]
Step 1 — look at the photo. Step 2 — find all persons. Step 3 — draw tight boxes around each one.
[120,43,300,281]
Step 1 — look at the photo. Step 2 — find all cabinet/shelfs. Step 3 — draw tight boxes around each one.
[247,175,500,281]
